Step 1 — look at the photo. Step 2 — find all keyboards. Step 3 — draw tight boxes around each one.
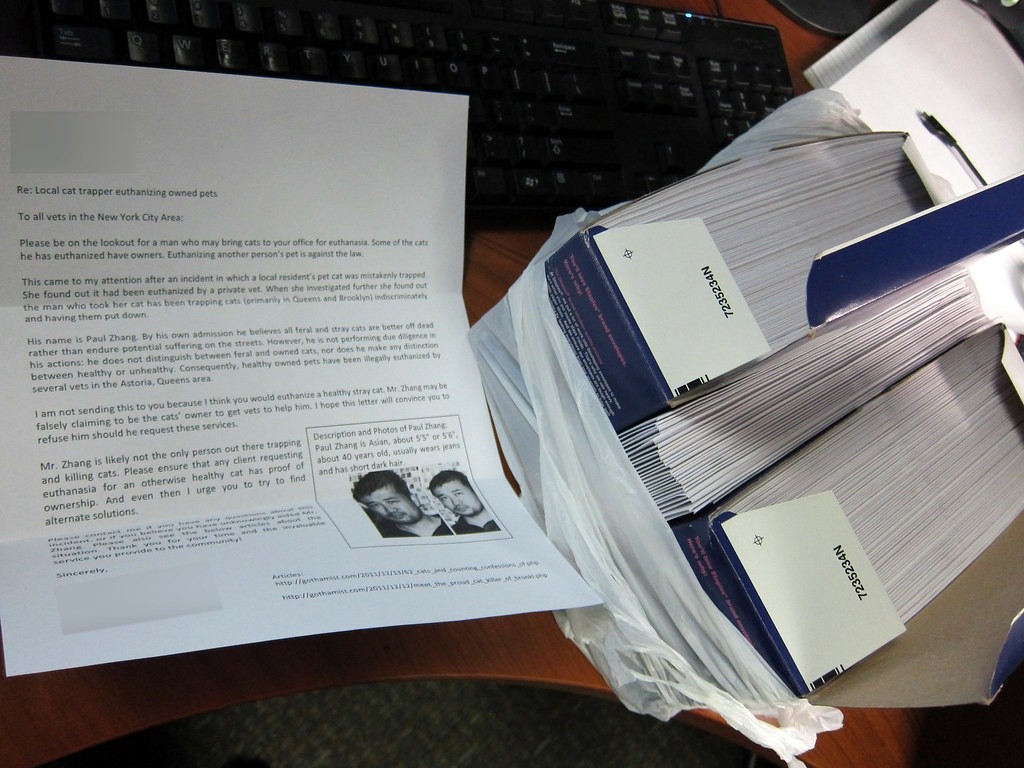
[0,0,799,230]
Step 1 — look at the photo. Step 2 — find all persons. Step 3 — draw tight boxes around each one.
[354,470,454,537]
[429,470,501,535]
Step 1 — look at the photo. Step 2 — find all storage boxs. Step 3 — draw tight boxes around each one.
[544,131,1024,436]
[668,323,1024,705]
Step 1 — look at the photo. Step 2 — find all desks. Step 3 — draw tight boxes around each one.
[1,0,1024,768]
